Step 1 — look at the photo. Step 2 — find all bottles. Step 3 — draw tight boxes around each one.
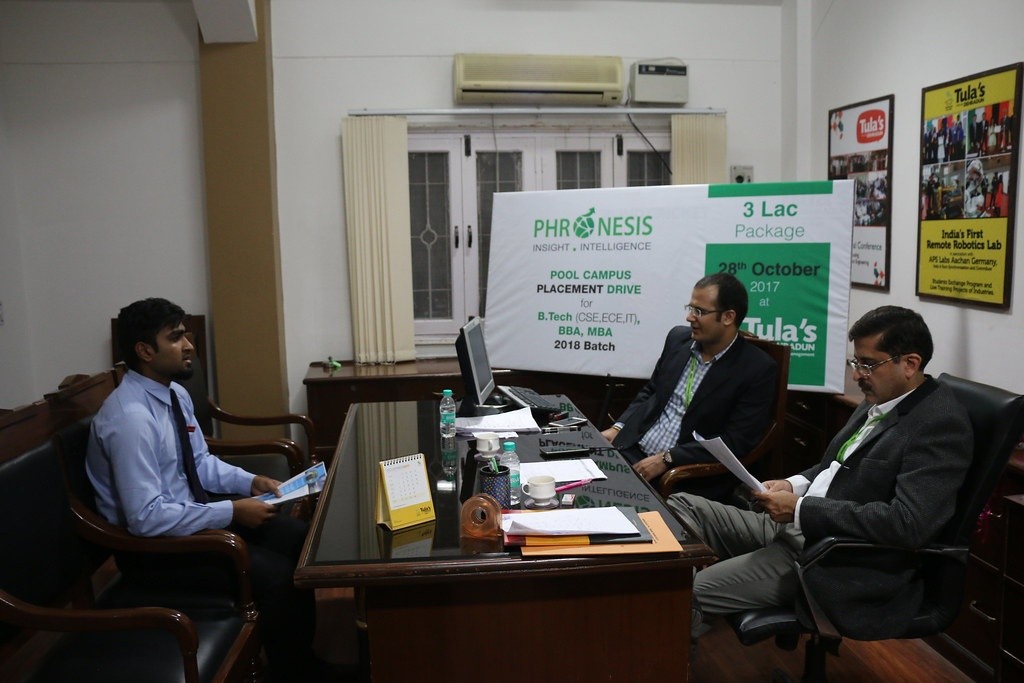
[440,437,457,481]
[501,442,521,506]
[439,390,456,439]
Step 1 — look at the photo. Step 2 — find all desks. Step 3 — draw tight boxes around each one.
[294,395,716,683]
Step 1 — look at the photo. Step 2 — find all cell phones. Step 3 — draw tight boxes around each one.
[548,417,588,427]
[539,446,590,456]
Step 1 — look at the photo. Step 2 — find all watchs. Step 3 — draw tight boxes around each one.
[662,448,674,470]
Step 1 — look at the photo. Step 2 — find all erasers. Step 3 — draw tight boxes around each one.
[561,494,575,505]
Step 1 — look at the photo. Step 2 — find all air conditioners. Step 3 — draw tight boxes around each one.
[452,53,624,107]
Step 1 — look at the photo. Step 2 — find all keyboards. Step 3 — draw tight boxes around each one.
[497,385,562,416]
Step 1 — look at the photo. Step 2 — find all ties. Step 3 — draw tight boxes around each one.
[169,387,209,506]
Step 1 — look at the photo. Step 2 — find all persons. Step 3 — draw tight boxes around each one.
[920,103,1015,222]
[827,149,888,228]
[597,273,780,508]
[85,298,319,683]
[665,303,975,648]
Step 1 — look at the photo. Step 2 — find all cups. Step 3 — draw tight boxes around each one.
[476,432,500,458]
[480,464,510,508]
[522,476,556,506]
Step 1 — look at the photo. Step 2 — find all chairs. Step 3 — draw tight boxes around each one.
[727,373,1024,683]
[0,362,315,683]
[608,329,791,503]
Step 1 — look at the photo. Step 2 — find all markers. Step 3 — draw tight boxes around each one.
[542,424,581,433]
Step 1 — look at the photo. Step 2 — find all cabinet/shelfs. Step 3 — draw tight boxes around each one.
[302,357,651,468]
[780,388,1024,683]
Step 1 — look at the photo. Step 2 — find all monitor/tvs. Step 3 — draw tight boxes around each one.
[454,316,500,418]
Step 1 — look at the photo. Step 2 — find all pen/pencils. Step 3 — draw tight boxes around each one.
[555,479,591,492]
[487,458,499,473]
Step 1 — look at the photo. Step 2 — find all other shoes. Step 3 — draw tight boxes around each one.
[322,659,361,683]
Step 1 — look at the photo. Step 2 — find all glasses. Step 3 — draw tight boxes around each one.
[845,356,900,376]
[682,303,723,317]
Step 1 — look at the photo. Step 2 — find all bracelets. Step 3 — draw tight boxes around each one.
[611,426,622,431]
